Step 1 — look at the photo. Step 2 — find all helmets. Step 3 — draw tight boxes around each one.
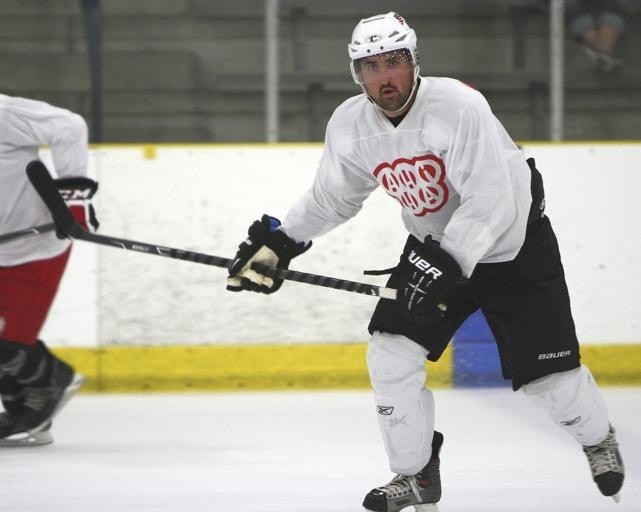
[347,11,421,86]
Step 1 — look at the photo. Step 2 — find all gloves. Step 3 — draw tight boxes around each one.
[53,177,101,241]
[225,212,313,295]
[396,233,464,330]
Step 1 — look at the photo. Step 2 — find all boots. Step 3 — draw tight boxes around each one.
[0,336,74,440]
[362,430,444,512]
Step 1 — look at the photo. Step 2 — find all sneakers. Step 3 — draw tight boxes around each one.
[582,421,626,498]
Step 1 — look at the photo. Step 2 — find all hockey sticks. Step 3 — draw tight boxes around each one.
[26,160,399,300]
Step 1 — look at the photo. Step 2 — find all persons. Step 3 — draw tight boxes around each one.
[0,93,97,448]
[226,12,623,512]
